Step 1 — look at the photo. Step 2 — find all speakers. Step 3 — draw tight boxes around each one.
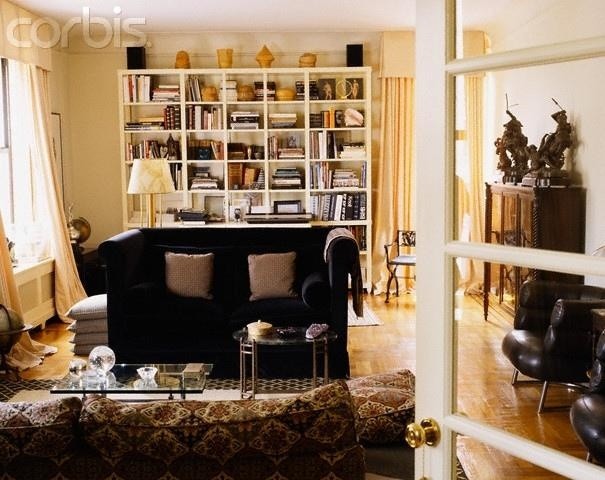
[125,46,147,70]
[346,43,364,68]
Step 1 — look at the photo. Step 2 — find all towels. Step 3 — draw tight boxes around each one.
[323,226,367,318]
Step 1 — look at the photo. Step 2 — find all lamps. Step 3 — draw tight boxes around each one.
[126,158,176,229]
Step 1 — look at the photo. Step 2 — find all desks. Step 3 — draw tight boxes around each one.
[589,308,604,383]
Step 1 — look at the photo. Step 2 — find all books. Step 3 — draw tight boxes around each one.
[349,225,367,250]
[310,161,365,187]
[125,139,159,160]
[168,164,183,189]
[188,138,223,160]
[293,79,320,99]
[185,77,202,101]
[252,81,275,100]
[189,166,220,188]
[124,75,182,101]
[310,193,366,221]
[267,113,297,127]
[310,131,365,159]
[186,105,222,129]
[267,135,304,160]
[173,206,210,227]
[309,105,335,128]
[126,105,181,131]
[228,140,247,159]
[218,79,238,103]
[270,166,303,190]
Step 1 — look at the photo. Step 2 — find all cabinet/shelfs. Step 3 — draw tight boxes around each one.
[116,66,374,295]
[481,180,588,322]
[12,258,58,332]
[72,246,101,293]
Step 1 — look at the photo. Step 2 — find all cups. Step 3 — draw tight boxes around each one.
[137,367,157,380]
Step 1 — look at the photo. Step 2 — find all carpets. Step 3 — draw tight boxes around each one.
[346,300,383,328]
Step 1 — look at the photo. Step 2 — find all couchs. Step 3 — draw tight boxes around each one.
[0,369,469,480]
[97,226,364,380]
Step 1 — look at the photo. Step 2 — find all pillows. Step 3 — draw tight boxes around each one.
[247,252,300,301]
[348,368,416,449]
[71,343,109,355]
[1,397,80,463]
[62,294,108,321]
[77,378,363,465]
[163,252,215,300]
[65,320,108,334]
[68,332,108,346]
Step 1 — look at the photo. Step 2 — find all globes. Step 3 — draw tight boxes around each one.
[67,217,92,254]
[0,304,34,383]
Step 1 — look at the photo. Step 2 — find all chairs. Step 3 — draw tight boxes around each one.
[383,230,417,304]
[500,279,605,415]
[569,330,605,467]
